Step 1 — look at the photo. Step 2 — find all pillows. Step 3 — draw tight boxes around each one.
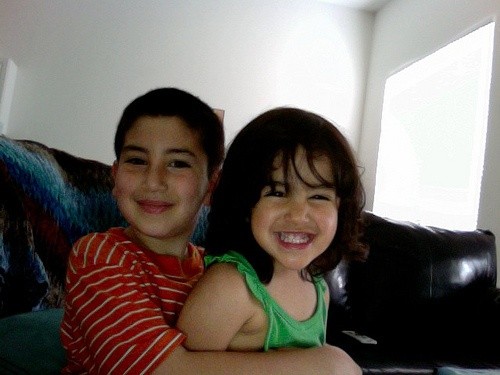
[0,307,67,375]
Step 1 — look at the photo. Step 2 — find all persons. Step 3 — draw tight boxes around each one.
[176,107,367,353]
[60,83,363,375]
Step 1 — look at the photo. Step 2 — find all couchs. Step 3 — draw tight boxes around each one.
[1,131,498,375]
